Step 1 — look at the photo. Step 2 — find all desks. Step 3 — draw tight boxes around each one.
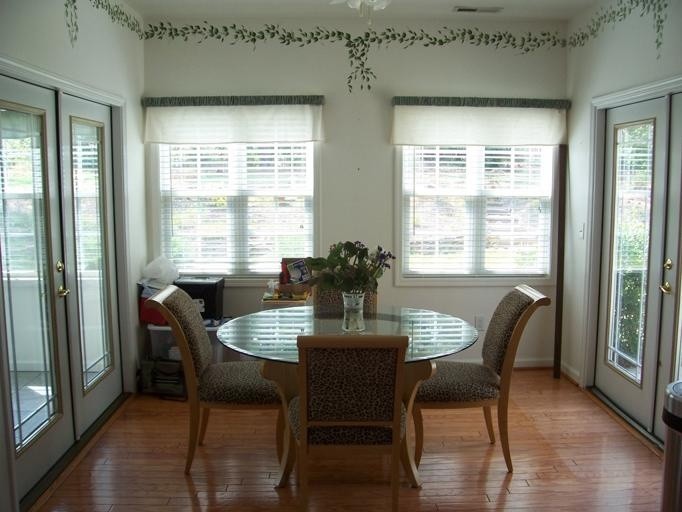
[216,304,479,490]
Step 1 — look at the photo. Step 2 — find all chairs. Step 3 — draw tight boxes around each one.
[287,334,409,496]
[403,282,552,474]
[311,278,380,308]
[142,283,286,475]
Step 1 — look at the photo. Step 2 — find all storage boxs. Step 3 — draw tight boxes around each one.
[140,276,229,362]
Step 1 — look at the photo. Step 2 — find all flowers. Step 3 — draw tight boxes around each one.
[302,238,398,328]
